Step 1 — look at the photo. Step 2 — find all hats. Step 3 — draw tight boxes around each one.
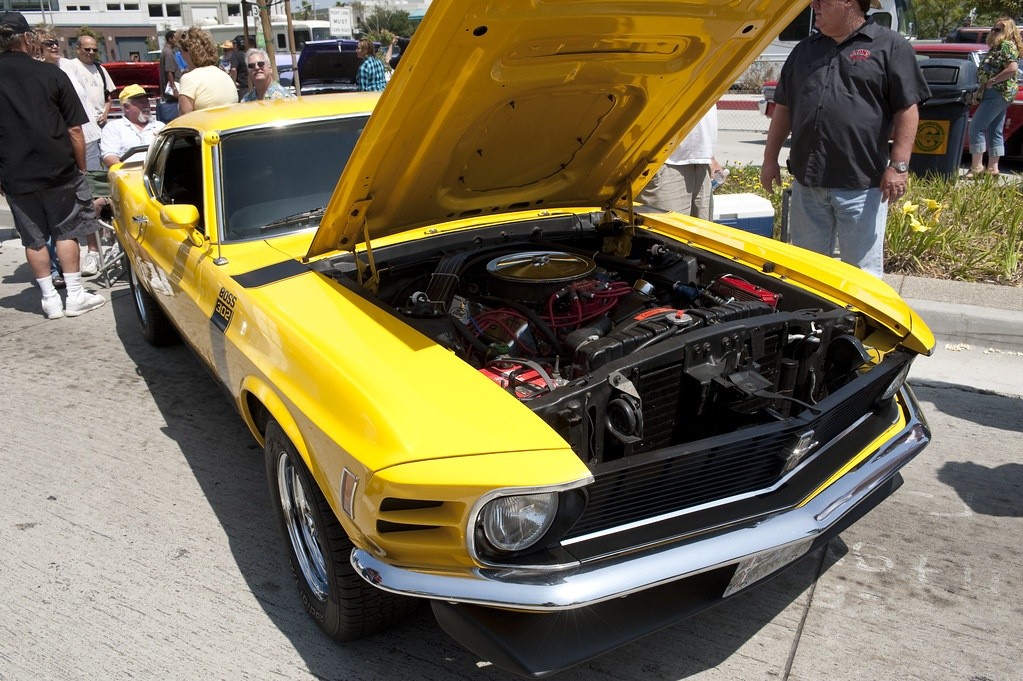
[0,10,36,36]
[870,0,881,10]
[220,41,233,48]
[119,83,155,105]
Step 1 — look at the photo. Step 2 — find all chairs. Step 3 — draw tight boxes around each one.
[86,169,133,287]
[167,142,206,231]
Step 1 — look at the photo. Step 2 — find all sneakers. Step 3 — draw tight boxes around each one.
[41,293,65,319]
[103,242,122,270]
[81,252,99,276]
[66,289,106,317]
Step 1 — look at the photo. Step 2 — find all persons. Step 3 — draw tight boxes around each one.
[960,17,1023,180]
[30,27,117,288]
[100,83,165,169]
[172,27,238,115]
[761,0,932,279]
[386,36,411,70]
[218,36,257,103]
[156,30,189,122]
[239,48,296,103]
[0,12,107,319]
[356,39,387,92]
[635,103,725,221]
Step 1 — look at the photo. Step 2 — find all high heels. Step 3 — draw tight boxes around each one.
[959,168,985,181]
[983,169,1000,179]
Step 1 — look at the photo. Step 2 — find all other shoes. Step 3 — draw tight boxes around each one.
[54,276,65,287]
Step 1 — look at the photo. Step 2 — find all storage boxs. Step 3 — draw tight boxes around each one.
[709,192,776,239]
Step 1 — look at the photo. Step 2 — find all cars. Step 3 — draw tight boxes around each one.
[758,22,1023,174]
[291,36,385,96]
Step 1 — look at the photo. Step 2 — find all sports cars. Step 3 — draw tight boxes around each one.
[101,60,163,121]
[105,1,942,643]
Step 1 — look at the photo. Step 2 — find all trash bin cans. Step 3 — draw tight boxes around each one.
[908,58,983,180]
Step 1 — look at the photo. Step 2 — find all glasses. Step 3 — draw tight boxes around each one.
[248,62,265,69]
[991,27,1002,33]
[41,39,59,47]
[223,49,232,52]
[80,46,99,53]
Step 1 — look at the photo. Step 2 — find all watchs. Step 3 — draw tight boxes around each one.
[992,77,996,85]
[890,161,909,172]
